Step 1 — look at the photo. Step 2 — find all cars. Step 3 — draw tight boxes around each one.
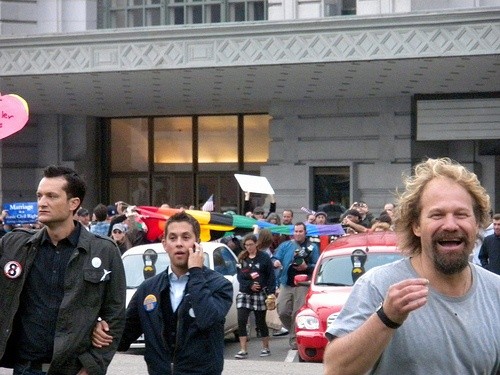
[293,230,416,364]
[97,240,250,340]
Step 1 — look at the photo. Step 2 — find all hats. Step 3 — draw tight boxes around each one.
[254,207,264,213]
[112,223,125,233]
[107,206,117,215]
[78,209,89,216]
[314,212,327,218]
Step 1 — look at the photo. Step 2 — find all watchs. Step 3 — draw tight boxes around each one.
[376,303,402,329]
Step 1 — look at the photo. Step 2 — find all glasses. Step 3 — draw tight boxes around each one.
[256,213,264,215]
[114,230,122,234]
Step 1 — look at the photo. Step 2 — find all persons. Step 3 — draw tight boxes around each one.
[0,165,126,375]
[469,209,494,266]
[340,200,396,235]
[271,210,340,348]
[94,211,233,375]
[1,191,276,254]
[234,234,276,359]
[324,156,500,375]
[477,214,500,276]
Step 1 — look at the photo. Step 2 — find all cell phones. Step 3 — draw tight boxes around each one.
[193,240,200,252]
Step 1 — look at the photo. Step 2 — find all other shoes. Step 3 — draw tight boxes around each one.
[290,342,298,350]
[273,328,288,336]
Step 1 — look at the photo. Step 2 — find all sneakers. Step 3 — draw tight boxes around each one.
[235,351,248,359]
[260,348,271,357]
